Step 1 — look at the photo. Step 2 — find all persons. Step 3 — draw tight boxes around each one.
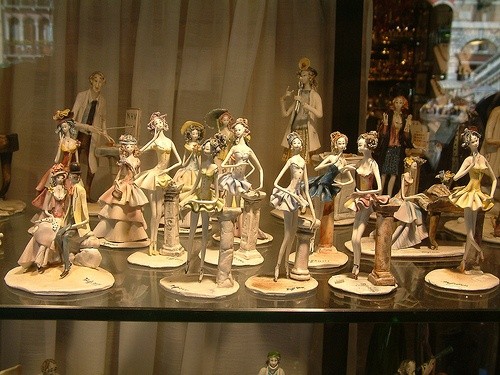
[20,59,500,375]
